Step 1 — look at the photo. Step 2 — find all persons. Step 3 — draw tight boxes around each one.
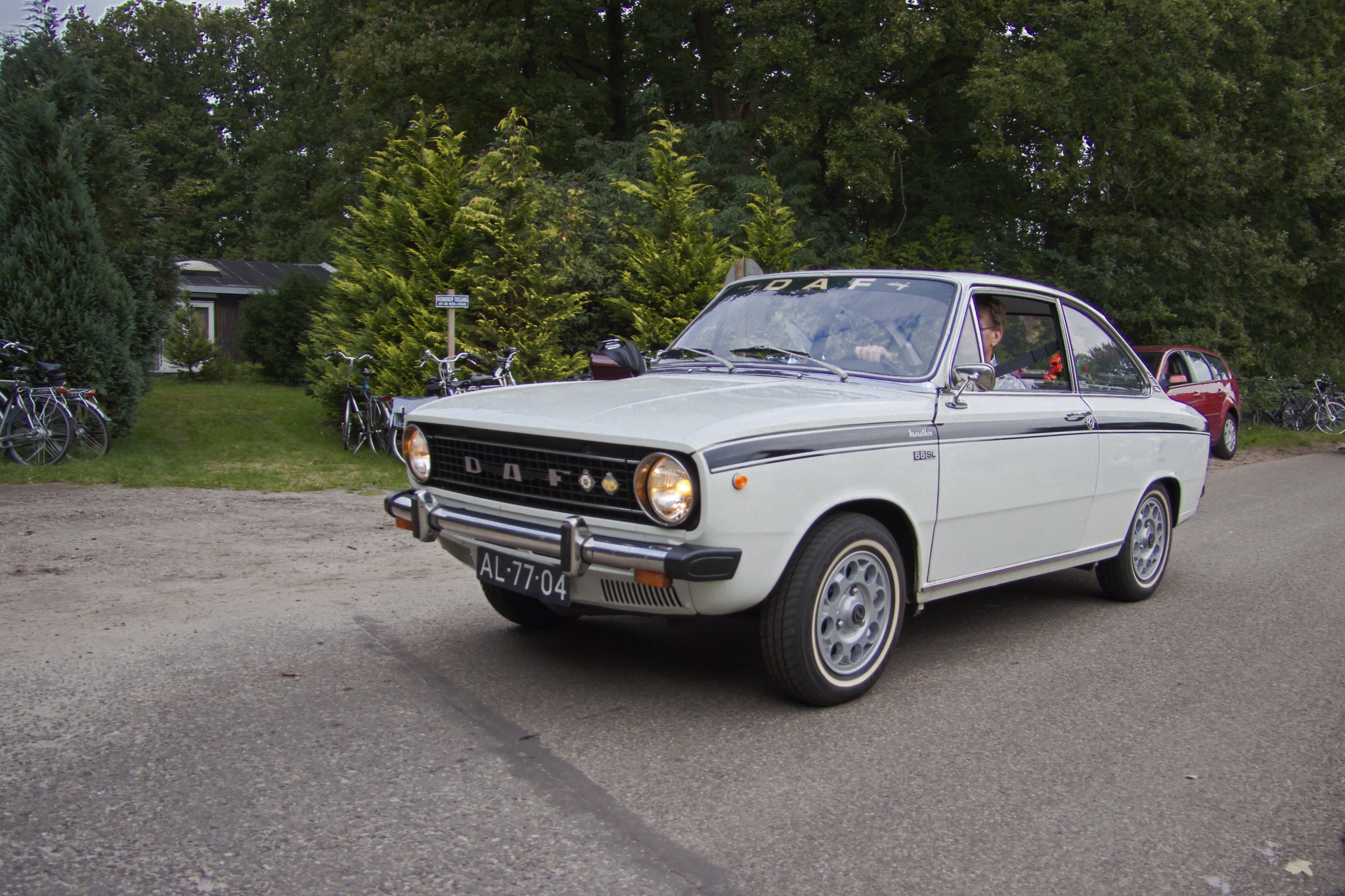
[851,296,1025,392]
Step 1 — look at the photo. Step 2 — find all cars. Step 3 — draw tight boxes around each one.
[378,265,1213,710]
[1129,343,1243,462]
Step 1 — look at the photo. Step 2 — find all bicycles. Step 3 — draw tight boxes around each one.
[322,346,523,466]
[0,337,114,468]
[1239,372,1345,436]
